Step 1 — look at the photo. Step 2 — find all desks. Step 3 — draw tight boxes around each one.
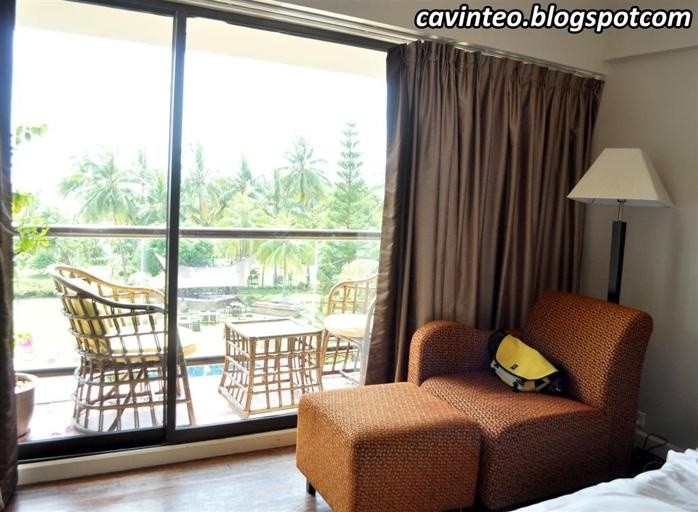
[218,316,324,419]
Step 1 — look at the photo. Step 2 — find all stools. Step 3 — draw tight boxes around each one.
[296,381,481,512]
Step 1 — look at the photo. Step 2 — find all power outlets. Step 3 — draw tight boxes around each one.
[636,411,646,428]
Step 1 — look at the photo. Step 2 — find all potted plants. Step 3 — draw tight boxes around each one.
[10,123,50,440]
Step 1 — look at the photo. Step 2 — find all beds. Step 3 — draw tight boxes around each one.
[510,449,698,512]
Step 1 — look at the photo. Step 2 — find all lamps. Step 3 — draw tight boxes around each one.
[566,146,676,304]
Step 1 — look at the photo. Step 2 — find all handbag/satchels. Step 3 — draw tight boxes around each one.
[490,333,565,391]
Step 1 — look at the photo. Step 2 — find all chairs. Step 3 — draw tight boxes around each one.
[47,263,196,434]
[406,291,654,512]
[320,273,378,386]
